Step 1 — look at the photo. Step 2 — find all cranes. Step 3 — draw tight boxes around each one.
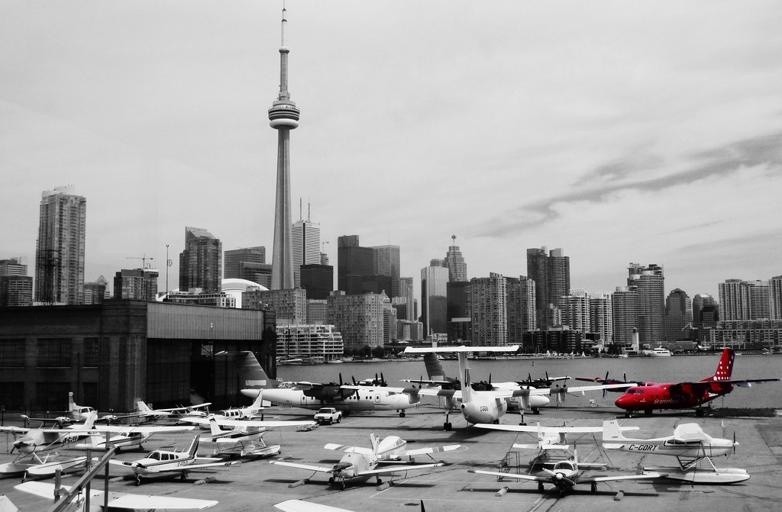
[125,253,155,268]
